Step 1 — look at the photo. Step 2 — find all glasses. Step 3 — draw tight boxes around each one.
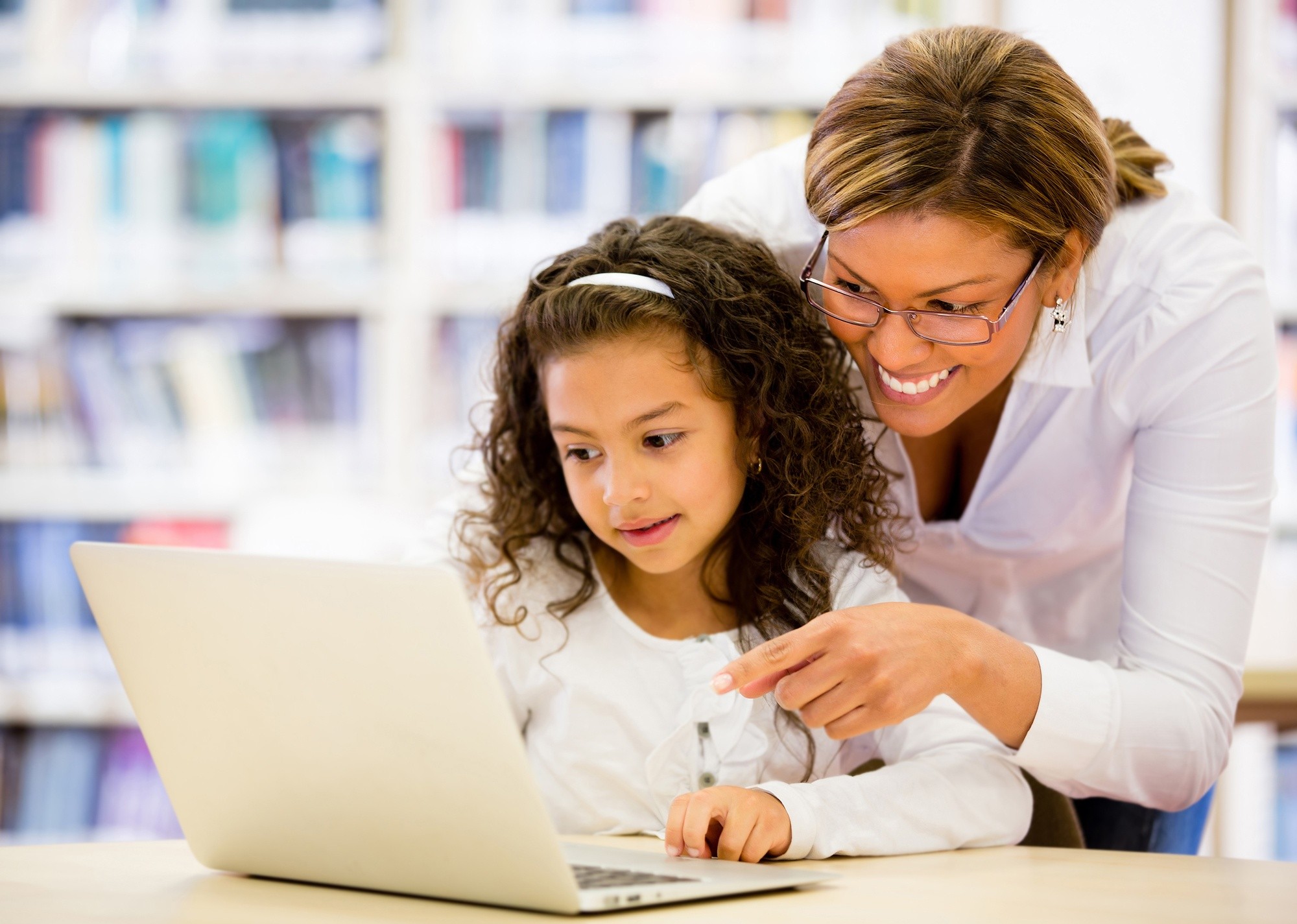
[797,223,1069,348]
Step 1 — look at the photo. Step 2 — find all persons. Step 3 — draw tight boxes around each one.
[433,214,1034,864]
[675,25,1277,855]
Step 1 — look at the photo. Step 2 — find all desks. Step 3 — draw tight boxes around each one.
[0,836,1297,924]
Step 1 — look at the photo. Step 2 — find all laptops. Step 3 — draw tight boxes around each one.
[66,539,840,914]
